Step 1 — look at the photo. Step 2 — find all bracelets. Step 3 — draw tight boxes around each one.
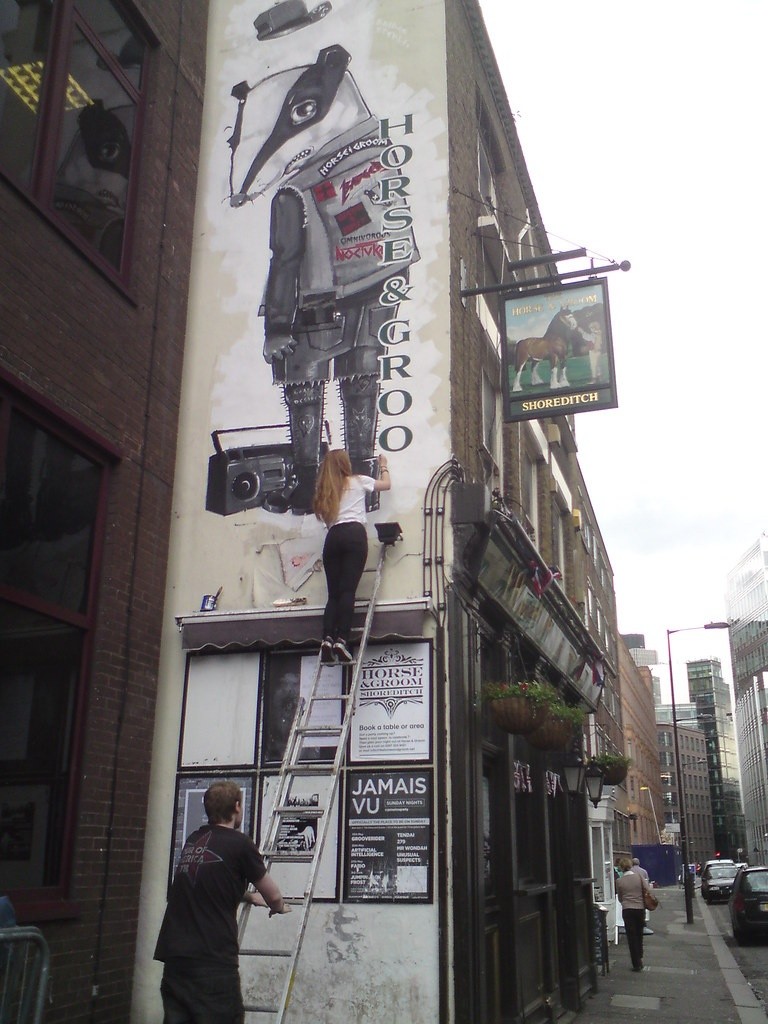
[381,465,387,468]
[382,470,388,474]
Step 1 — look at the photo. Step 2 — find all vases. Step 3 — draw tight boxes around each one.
[604,766,630,784]
[532,719,570,749]
[491,698,548,733]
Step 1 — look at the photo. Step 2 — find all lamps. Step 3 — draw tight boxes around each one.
[374,522,403,544]
[544,740,604,809]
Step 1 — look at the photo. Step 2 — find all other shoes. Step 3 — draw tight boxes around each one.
[632,962,644,971]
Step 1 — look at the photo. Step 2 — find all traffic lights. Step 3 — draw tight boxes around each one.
[715,852,720,857]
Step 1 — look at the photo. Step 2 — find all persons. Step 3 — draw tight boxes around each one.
[153,780,292,1024]
[631,858,654,934]
[616,858,650,972]
[312,448,390,667]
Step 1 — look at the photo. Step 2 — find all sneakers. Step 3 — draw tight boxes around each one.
[319,635,335,667]
[332,637,353,662]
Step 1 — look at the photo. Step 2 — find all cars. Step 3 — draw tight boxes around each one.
[734,863,748,869]
[700,866,739,904]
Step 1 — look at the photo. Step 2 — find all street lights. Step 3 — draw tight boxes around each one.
[665,622,731,927]
[639,786,662,844]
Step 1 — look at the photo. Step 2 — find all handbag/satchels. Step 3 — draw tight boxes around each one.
[639,874,658,911]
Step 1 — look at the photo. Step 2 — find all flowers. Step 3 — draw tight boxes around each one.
[480,682,586,725]
[587,752,631,771]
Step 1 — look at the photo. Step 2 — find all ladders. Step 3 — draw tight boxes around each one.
[235,541,388,1024]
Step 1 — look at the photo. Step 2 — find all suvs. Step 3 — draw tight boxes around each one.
[723,865,768,946]
[703,859,734,870]
